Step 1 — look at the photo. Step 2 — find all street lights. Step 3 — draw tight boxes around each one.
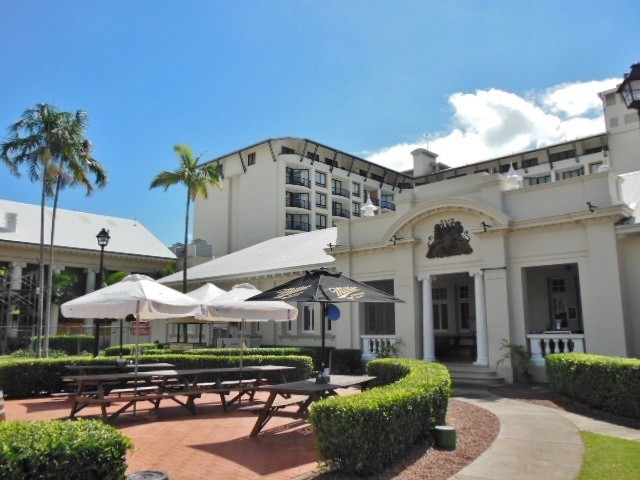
[94,228,111,357]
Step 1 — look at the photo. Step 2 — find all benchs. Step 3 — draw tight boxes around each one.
[50,386,160,397]
[179,378,256,390]
[77,390,202,406]
[238,397,308,428]
[206,384,273,397]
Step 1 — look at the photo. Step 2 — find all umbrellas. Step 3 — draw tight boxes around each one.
[194,283,298,348]
[242,267,406,372]
[184,282,229,349]
[60,273,201,416]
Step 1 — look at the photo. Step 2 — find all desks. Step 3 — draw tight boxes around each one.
[62,368,208,425]
[191,365,296,413]
[249,374,377,437]
[65,362,175,374]
